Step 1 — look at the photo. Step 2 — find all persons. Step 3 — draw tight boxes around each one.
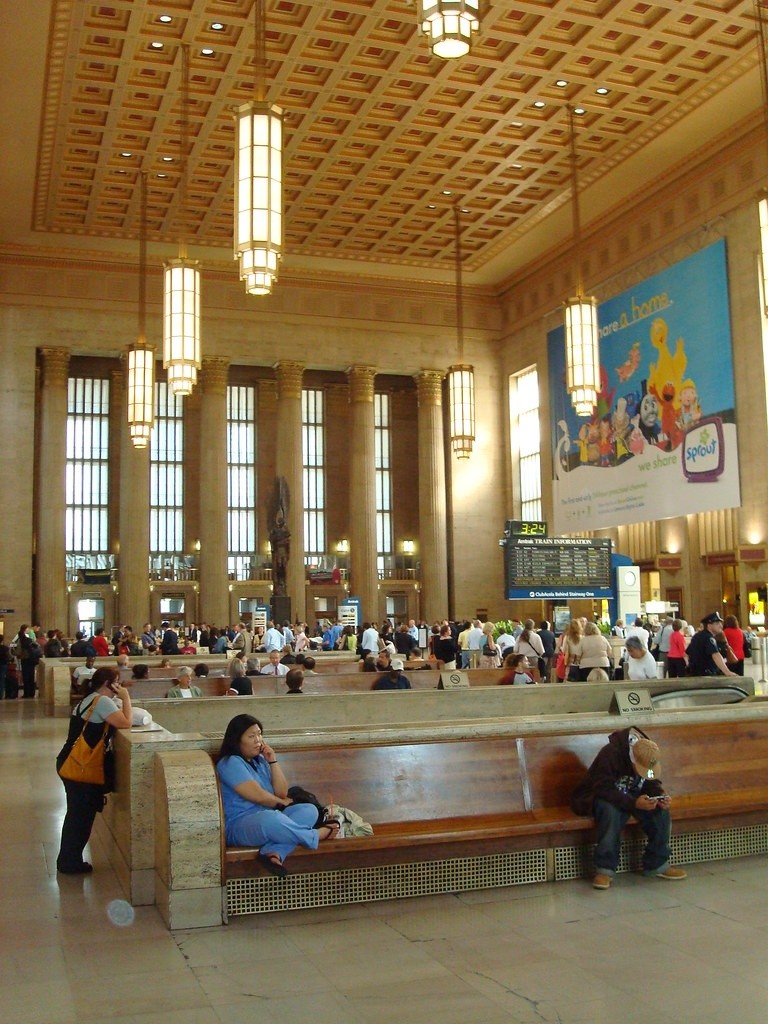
[213,714,340,876]
[571,726,688,888]
[0,608,754,702]
[57,668,133,873]
[270,518,290,596]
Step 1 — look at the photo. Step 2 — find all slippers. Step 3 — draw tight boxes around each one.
[316,820,340,840]
[256,855,287,877]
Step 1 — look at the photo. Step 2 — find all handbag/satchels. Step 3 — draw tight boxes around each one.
[538,658,545,670]
[483,635,497,656]
[651,644,660,661]
[743,634,752,658]
[58,734,106,785]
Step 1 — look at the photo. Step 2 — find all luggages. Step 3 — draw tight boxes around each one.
[5,661,18,698]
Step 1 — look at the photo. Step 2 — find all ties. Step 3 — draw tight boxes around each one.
[275,667,278,675]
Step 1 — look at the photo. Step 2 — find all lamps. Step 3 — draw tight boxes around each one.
[161,39,202,396]
[563,102,603,417]
[127,170,159,449]
[447,205,475,458]
[231,0,289,296]
[417,0,481,61]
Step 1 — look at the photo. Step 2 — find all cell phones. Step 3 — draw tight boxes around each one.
[647,795,670,801]
[108,681,119,694]
[259,743,265,751]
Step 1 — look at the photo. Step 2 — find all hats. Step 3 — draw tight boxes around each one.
[700,612,724,624]
[390,659,403,671]
[633,738,662,780]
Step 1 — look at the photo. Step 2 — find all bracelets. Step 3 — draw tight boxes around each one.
[268,761,277,764]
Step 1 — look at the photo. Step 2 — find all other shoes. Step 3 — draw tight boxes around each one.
[57,862,92,873]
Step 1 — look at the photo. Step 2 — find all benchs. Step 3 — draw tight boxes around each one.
[38,635,768,929]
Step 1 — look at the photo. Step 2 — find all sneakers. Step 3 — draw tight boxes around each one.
[656,867,687,880]
[593,873,613,888]
